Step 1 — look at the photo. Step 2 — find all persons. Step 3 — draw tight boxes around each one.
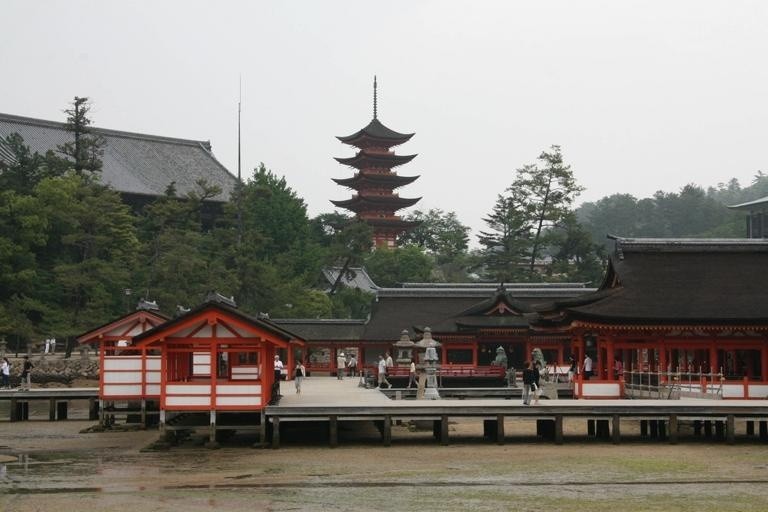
[406,358,419,388]
[274,355,283,387]
[51,336,56,355]
[522,359,543,406]
[292,359,306,394]
[385,351,393,367]
[375,354,393,389]
[0,357,12,389]
[21,356,35,390]
[580,352,593,380]
[44,337,50,355]
[347,353,357,377]
[567,353,576,383]
[615,357,623,380]
[337,353,346,379]
